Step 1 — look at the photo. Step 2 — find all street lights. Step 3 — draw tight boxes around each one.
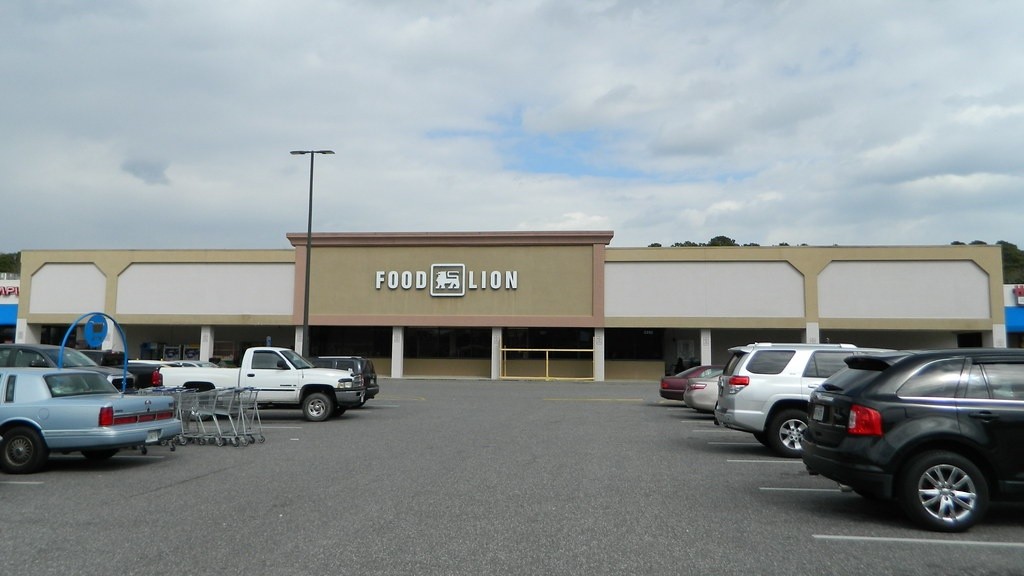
[291,147,339,362]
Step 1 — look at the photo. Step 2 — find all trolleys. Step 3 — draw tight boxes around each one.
[135,383,267,448]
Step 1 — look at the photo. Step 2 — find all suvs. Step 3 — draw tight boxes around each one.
[713,342,907,458]
[310,354,379,402]
[801,346,1024,532]
[0,343,137,399]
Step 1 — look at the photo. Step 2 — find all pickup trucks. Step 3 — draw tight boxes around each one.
[153,346,365,422]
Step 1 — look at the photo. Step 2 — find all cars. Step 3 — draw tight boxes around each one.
[680,374,720,415]
[122,356,222,390]
[658,364,729,401]
[0,366,182,471]
